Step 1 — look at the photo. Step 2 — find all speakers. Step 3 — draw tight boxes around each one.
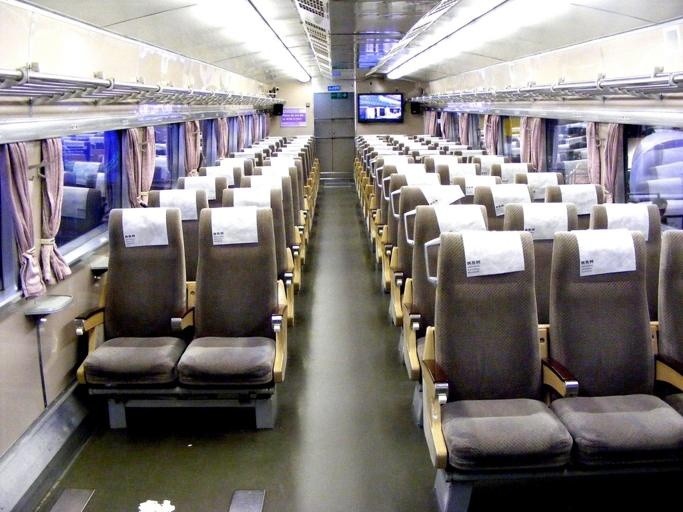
[273,104,283,115]
[411,102,420,115]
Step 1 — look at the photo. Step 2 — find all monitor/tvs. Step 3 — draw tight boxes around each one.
[358,93,404,123]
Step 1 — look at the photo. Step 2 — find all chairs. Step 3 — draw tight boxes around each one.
[347,130,681,512]
[68,131,323,434]
